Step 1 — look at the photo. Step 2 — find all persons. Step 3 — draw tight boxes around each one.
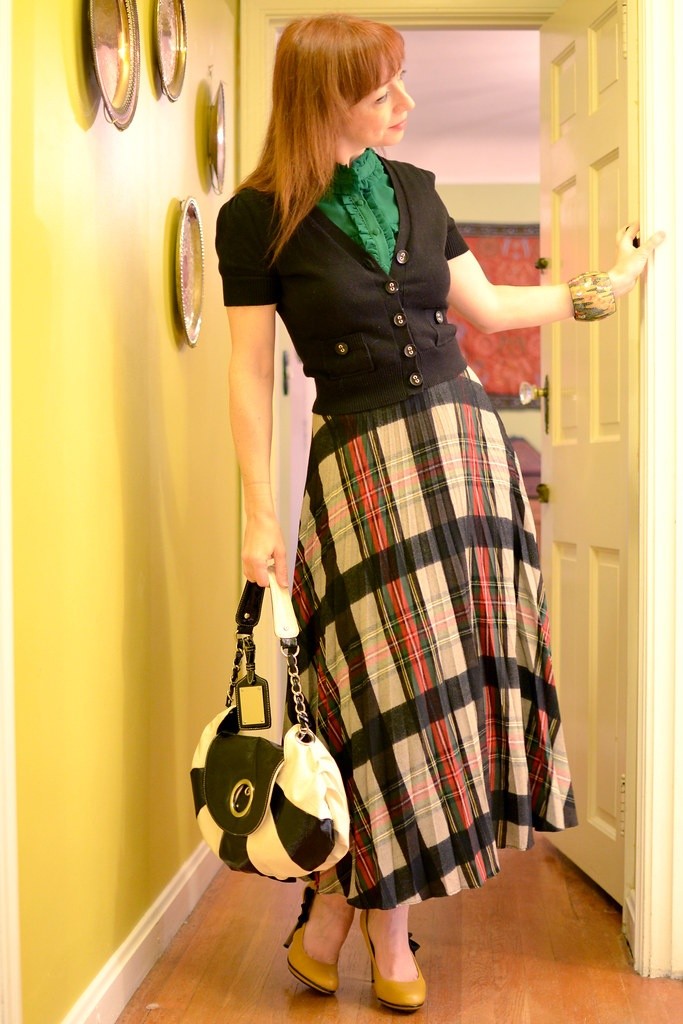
[214,13,665,1014]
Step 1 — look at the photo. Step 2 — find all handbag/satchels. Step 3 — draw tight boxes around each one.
[189,570,350,883]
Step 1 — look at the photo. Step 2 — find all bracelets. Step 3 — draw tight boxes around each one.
[568,272,617,320]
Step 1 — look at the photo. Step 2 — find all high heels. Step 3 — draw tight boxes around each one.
[358,906,427,1011]
[282,886,342,996]
